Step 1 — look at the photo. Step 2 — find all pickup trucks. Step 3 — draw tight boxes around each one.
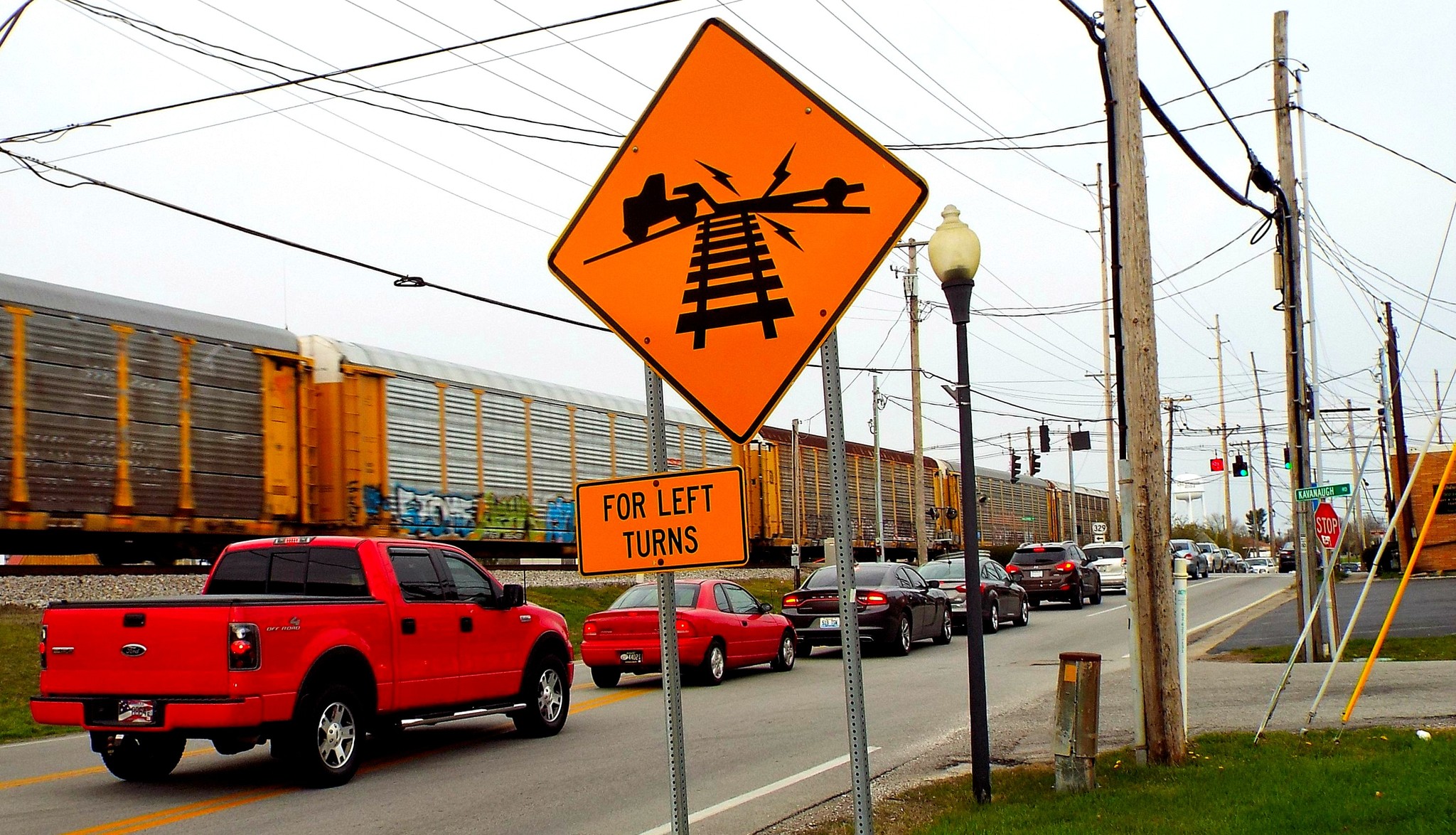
[28,535,575,790]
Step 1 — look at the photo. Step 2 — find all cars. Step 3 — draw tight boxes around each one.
[1233,552,1279,574]
[1277,542,1323,574]
[1170,539,1209,581]
[782,563,954,657]
[581,577,799,689]
[1169,540,1184,585]
[1325,548,1361,574]
[915,556,1029,634]
[1196,541,1224,573]
[1220,548,1237,573]
[1080,542,1126,593]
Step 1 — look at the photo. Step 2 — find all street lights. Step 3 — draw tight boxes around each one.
[927,205,996,808]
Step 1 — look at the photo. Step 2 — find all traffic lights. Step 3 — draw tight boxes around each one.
[1377,399,1388,434]
[1233,455,1248,478]
[1307,386,1315,419]
[1030,453,1041,476]
[1010,456,1022,484]
[1284,448,1291,469]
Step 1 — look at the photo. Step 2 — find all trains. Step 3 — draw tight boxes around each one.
[0,271,1121,574]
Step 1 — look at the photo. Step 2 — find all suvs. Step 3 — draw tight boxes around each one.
[1004,540,1102,610]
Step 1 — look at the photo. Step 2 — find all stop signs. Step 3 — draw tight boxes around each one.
[1313,502,1341,550]
